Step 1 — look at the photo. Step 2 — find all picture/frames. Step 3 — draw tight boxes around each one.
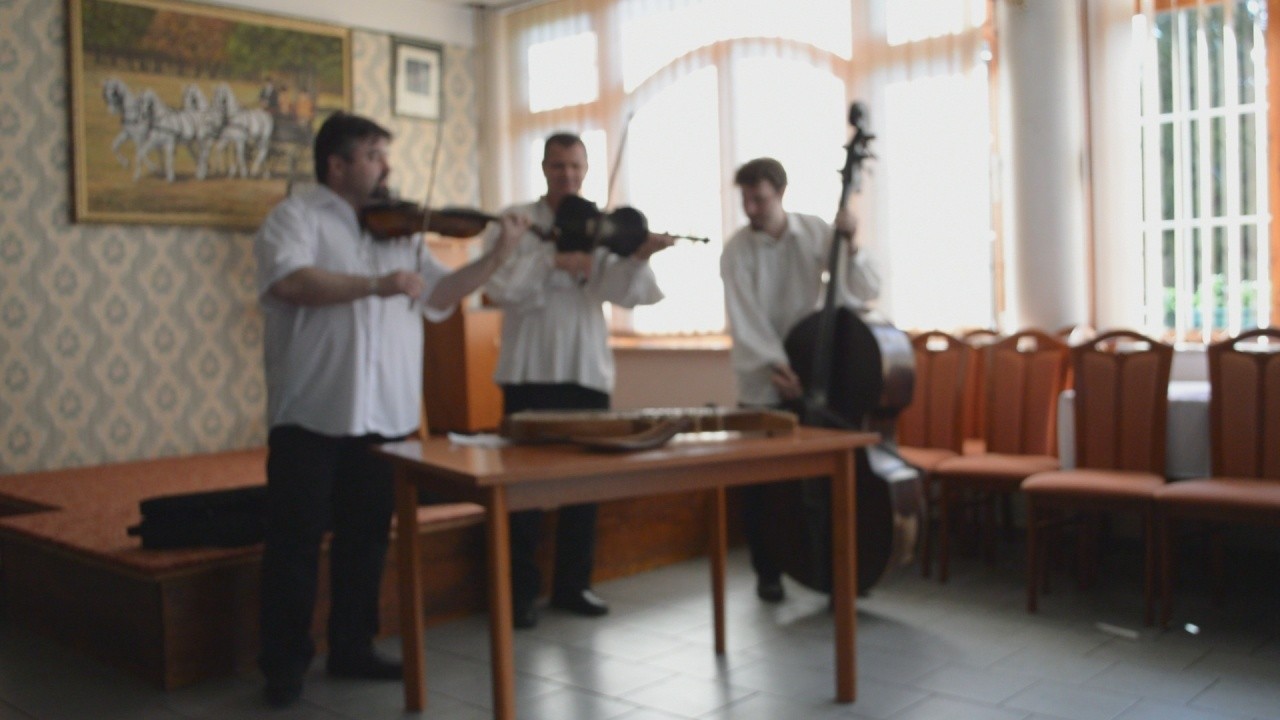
[68,0,352,227]
[389,36,444,120]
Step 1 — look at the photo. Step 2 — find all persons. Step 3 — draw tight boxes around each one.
[718,158,884,602]
[252,109,534,705]
[481,134,678,631]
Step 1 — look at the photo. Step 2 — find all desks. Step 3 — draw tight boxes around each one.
[370,425,883,720]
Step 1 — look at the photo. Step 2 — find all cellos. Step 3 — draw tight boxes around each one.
[755,101,924,596]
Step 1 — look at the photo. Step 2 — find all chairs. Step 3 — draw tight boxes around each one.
[894,327,1280,626]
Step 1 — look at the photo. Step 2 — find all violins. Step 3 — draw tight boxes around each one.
[556,194,709,258]
[366,201,561,241]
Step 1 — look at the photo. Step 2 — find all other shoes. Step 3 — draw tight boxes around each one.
[331,654,403,680]
[271,682,305,708]
[515,600,537,632]
[757,578,784,602]
[552,589,609,615]
